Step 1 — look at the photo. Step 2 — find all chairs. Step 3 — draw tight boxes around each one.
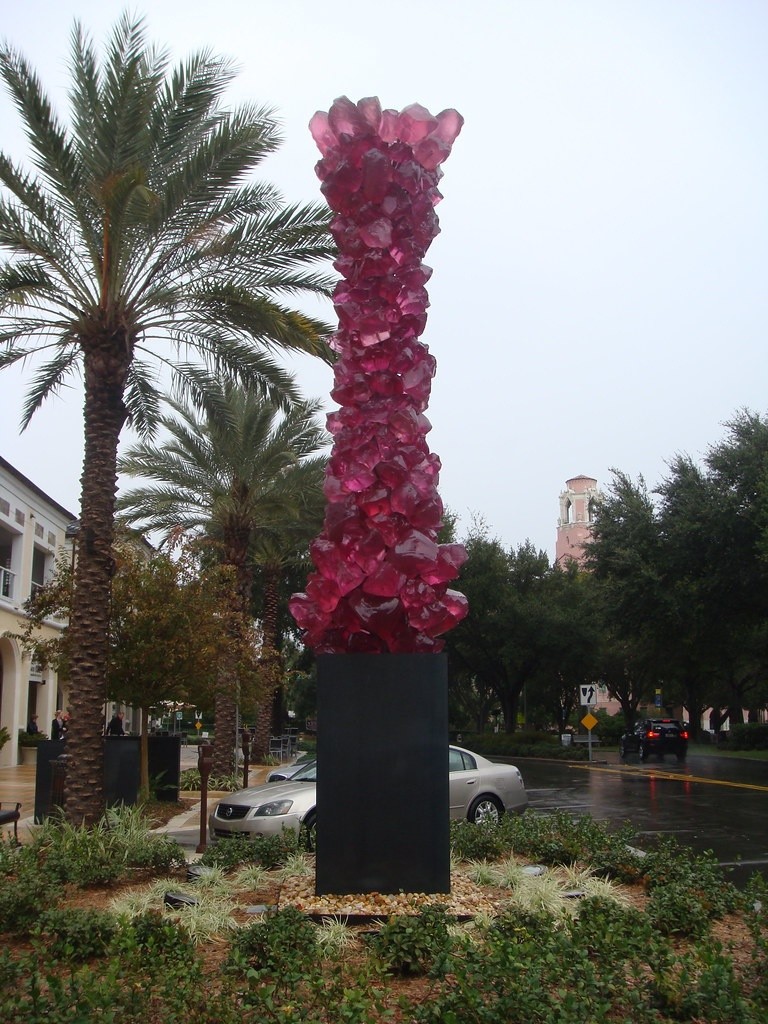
[267,733,298,764]
[1,802,22,847]
[155,731,188,747]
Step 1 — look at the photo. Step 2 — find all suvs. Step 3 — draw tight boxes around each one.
[618,719,688,761]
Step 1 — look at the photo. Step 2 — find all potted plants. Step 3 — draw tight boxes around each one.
[19,729,49,764]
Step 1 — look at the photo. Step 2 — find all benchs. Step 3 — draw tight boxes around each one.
[573,735,602,746]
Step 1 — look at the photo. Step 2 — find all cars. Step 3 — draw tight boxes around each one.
[265,764,307,783]
[208,745,529,852]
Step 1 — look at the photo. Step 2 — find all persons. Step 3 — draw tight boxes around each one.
[51,710,69,740]
[26,714,43,736]
[104,711,125,737]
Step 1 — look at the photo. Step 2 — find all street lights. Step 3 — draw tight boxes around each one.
[287,707,295,756]
